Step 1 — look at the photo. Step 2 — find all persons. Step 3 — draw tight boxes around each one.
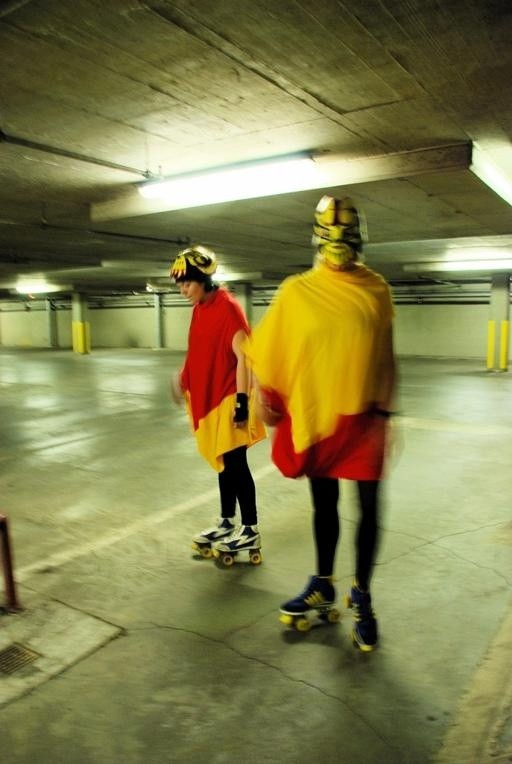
[239,193,401,654]
[169,245,268,554]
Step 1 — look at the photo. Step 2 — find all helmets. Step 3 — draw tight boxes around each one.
[309,195,369,251]
[170,246,217,279]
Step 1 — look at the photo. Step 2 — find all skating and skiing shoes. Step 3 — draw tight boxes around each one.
[193,516,261,566]
[279,576,339,630]
[347,585,379,652]
